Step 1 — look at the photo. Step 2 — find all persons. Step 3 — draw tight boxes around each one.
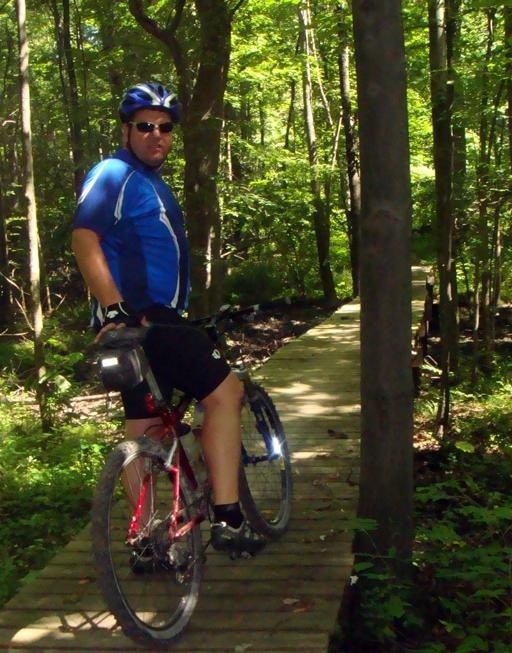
[71,82,267,574]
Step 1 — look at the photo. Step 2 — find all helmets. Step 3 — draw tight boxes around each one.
[118,81,181,123]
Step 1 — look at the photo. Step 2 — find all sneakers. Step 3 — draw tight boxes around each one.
[209,515,268,552]
[128,546,208,575]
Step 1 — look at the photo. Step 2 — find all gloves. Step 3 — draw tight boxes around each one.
[100,299,145,330]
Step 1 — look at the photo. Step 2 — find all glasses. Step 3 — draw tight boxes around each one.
[126,119,174,133]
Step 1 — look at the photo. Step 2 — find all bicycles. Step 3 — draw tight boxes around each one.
[92,293,295,645]
